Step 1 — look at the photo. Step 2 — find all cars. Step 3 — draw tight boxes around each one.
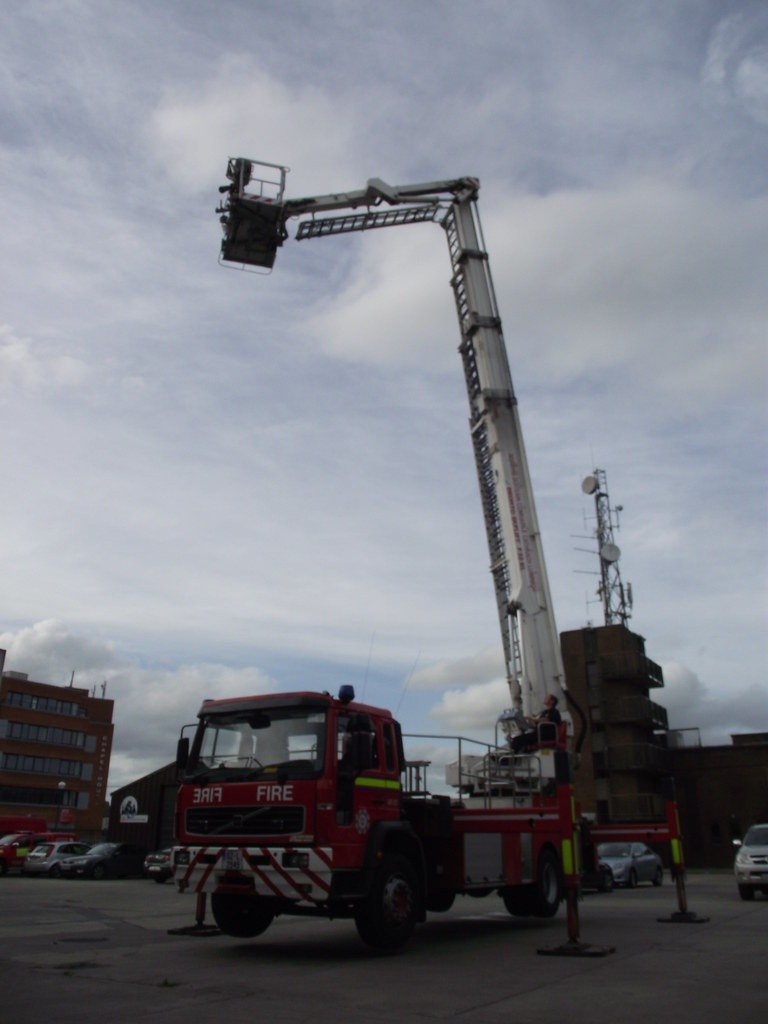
[594,841,665,892]
[24,840,93,879]
[58,841,154,882]
[144,847,175,884]
[731,824,768,899]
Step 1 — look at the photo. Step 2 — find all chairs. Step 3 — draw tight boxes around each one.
[263,741,290,766]
[337,733,373,786]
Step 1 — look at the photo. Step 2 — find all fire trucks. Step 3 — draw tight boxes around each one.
[167,155,674,943]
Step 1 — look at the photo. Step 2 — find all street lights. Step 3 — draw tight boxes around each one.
[51,781,68,834]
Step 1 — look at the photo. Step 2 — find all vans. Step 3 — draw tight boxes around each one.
[0,831,78,876]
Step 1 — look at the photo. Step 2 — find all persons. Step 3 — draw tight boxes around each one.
[509,694,562,764]
